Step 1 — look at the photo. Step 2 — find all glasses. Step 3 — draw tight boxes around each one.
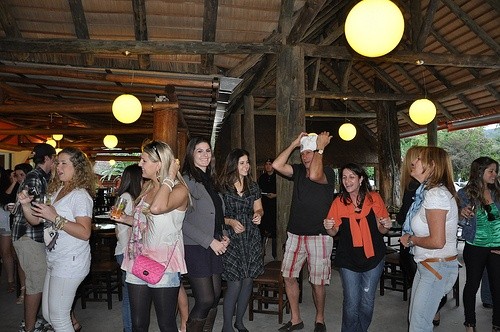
[148,140,161,161]
[484,204,494,221]
[47,154,57,159]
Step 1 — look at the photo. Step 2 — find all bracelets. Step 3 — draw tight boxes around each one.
[162,176,175,193]
[55,216,68,230]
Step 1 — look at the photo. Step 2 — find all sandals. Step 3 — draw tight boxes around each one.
[72,321,82,331]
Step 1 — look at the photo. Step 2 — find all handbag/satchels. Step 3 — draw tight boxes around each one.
[132,256,165,284]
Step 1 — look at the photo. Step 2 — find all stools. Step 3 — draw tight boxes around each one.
[249,260,304,322]
[80,187,125,309]
[376,210,462,308]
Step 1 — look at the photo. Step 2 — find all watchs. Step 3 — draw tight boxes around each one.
[407,235,415,247]
[313,149,323,155]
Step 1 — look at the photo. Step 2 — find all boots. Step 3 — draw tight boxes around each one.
[186,317,205,332]
[203,308,218,332]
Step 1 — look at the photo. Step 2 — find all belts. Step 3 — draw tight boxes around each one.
[419,255,457,280]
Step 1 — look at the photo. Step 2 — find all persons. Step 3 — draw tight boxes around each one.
[323,163,393,332]
[396,146,447,326]
[481,177,500,307]
[206,149,264,332]
[258,159,277,264]
[457,156,500,332]
[0,163,34,305]
[272,131,336,332]
[117,140,192,332]
[10,143,57,332]
[400,146,462,332]
[179,135,230,332]
[115,164,152,332]
[31,147,95,332]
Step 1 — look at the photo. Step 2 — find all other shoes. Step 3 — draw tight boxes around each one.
[314,321,326,332]
[432,311,440,326]
[19,318,55,332]
[9,288,16,294]
[279,321,303,332]
[16,286,25,304]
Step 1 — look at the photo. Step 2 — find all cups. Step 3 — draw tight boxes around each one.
[110,197,127,219]
[40,193,51,222]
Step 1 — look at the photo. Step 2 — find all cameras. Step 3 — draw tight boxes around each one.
[28,187,40,196]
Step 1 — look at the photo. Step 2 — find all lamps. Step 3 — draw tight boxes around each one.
[409,64,437,127]
[46,133,64,155]
[345,0,405,58]
[103,134,118,149]
[338,99,356,141]
[112,60,142,124]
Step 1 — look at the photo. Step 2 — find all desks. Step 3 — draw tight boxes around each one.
[92,223,116,232]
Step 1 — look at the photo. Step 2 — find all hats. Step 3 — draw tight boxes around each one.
[299,133,319,153]
[265,158,274,163]
